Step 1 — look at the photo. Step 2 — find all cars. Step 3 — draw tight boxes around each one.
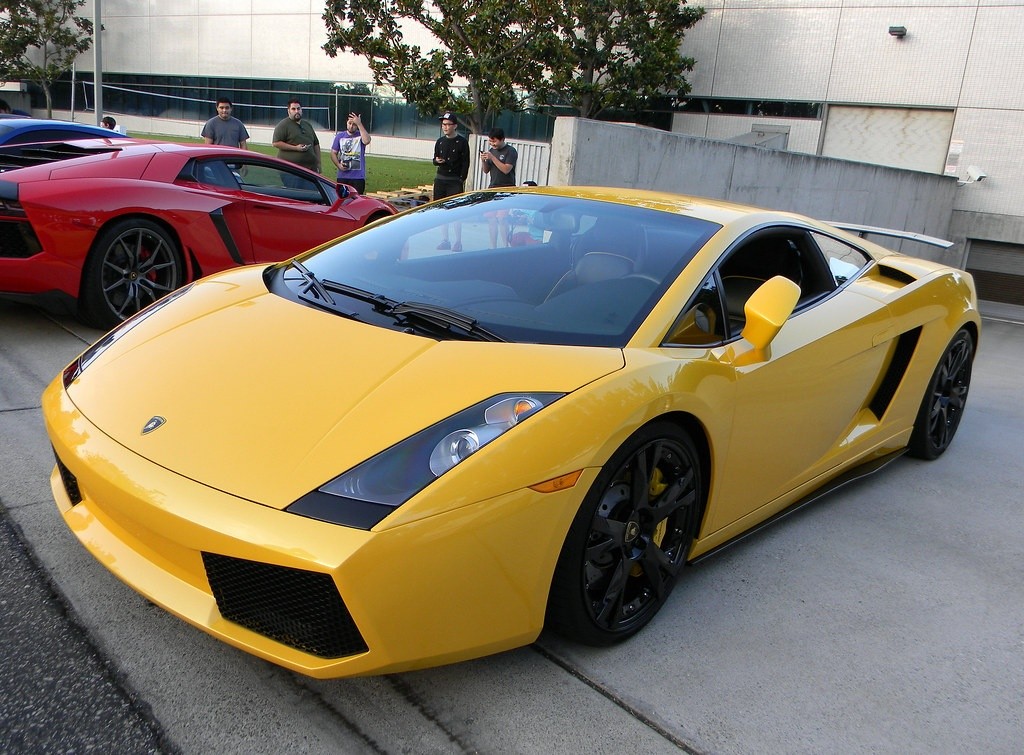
[0,113,133,174]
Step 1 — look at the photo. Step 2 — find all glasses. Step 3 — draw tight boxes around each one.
[300,125,305,134]
[442,123,454,127]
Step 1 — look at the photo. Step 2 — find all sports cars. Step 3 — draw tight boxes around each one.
[0,138,402,331]
[39,184,983,681]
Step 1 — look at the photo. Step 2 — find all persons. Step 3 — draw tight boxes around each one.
[432,113,470,252]
[481,128,518,249]
[201,97,250,176]
[0,99,31,116]
[271,98,322,190]
[331,112,371,195]
[100,117,116,130]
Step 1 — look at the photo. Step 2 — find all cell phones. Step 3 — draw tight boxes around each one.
[480,151,483,154]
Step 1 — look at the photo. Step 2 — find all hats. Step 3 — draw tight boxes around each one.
[439,111,457,124]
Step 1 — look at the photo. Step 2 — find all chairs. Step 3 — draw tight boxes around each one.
[544,250,636,306]
[715,276,766,331]
